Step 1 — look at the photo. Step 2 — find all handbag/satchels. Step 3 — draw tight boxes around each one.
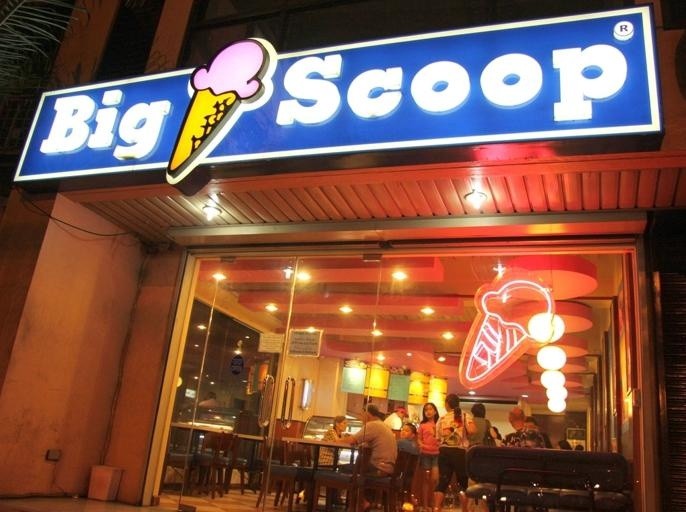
[483,434,495,446]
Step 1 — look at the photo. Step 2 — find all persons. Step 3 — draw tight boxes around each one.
[318,392,585,511]
[199,390,221,408]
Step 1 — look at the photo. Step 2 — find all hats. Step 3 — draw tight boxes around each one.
[397,407,408,418]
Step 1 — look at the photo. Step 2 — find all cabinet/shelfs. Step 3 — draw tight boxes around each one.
[300,416,362,464]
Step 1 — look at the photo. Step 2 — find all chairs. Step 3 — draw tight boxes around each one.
[158,422,418,511]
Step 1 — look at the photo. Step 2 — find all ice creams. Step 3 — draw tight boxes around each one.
[168,38,269,176]
[466,279,551,383]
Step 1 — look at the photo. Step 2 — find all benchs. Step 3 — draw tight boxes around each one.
[464,443,633,511]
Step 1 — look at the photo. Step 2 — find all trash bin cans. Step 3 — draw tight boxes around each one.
[88,464,122,501]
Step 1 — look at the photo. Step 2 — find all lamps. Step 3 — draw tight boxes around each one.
[529,313,569,415]
[339,359,448,410]
